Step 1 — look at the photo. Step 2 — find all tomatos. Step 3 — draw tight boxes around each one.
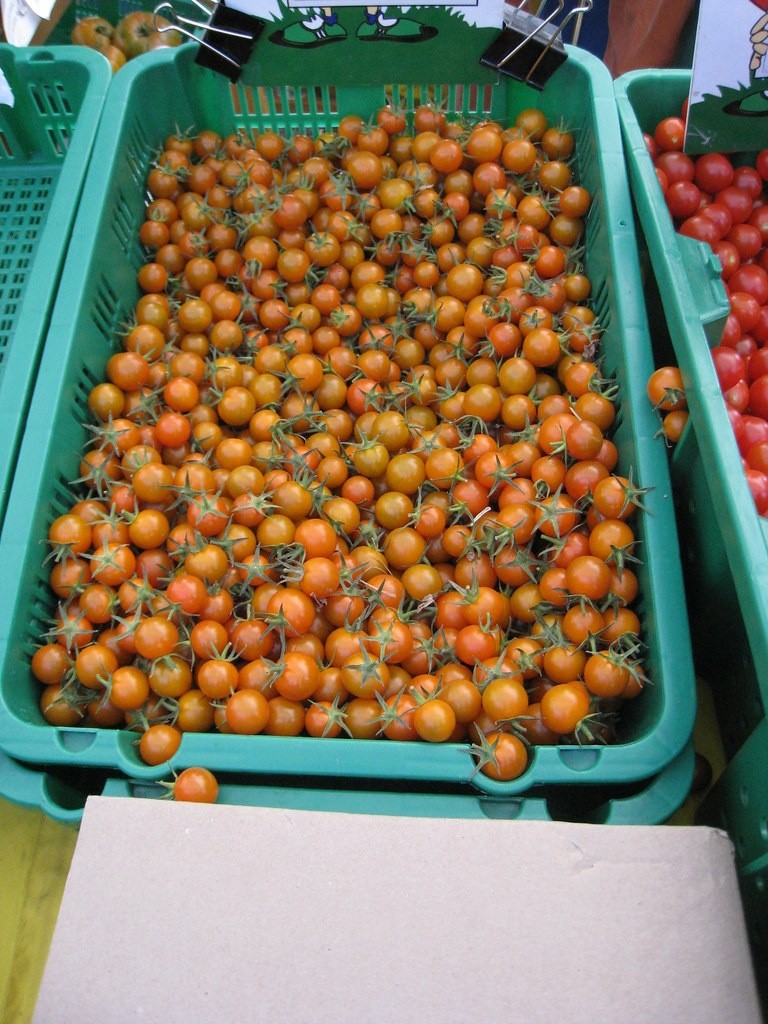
[32,101,652,805]
[72,10,184,70]
[637,93,767,519]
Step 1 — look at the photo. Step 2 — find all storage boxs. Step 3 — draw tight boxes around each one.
[0,0,768,933]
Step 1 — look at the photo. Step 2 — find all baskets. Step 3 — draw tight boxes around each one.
[0,1,768,826]
